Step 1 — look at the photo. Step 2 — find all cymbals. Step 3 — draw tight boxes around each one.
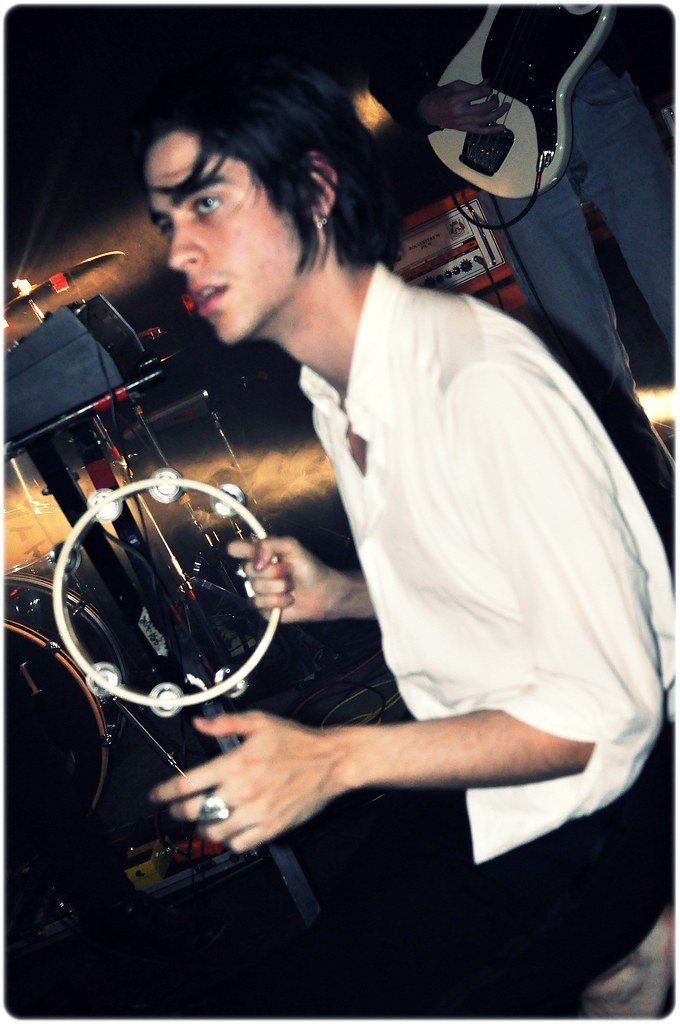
[51,466,284,718]
[4,251,127,315]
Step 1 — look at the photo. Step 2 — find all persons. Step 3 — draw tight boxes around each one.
[136,68,674,1020]
[369,4,676,566]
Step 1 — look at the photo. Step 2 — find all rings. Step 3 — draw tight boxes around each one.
[206,795,229,820]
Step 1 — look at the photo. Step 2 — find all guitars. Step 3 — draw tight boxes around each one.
[427,5,616,201]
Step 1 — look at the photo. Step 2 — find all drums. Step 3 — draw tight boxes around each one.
[5,570,126,839]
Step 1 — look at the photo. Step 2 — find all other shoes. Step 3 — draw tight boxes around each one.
[581,908,672,1018]
